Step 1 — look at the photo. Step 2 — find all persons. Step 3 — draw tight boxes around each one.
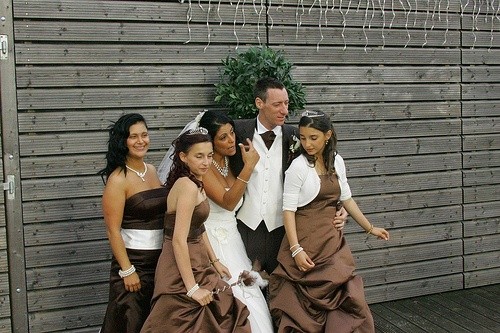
[136,128,253,333]
[97,112,170,333]
[158,108,278,333]
[215,75,348,305]
[261,107,390,333]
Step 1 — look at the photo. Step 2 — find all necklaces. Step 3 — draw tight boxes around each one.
[124,160,148,182]
[211,155,230,177]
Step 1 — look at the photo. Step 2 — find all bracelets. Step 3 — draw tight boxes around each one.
[210,258,219,264]
[119,265,136,278]
[186,284,199,298]
[367,225,373,235]
[236,177,248,184]
[289,244,304,258]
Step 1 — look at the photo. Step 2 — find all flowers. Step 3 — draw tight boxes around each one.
[211,269,269,298]
[289,135,302,157]
